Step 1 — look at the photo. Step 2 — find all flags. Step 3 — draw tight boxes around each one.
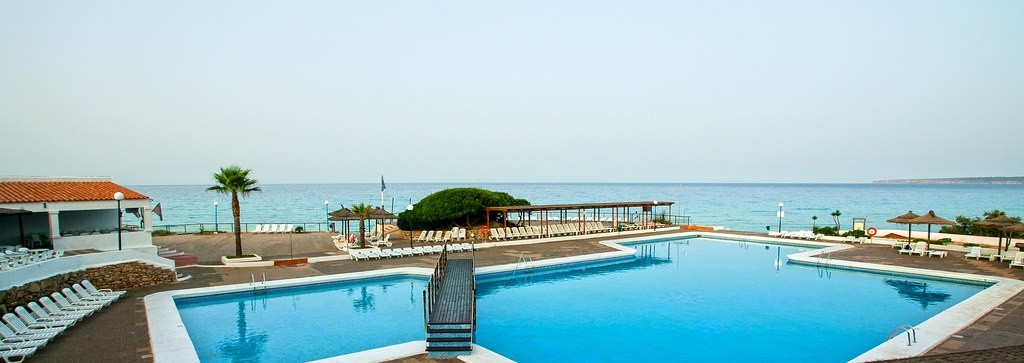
[153,203,163,222]
[381,176,386,191]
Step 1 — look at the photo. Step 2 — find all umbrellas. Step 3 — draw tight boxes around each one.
[886,279,950,308]
[973,212,1024,261]
[887,211,920,244]
[328,205,400,246]
[904,210,957,256]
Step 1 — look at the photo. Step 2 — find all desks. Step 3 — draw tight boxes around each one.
[26,249,49,255]
[930,251,947,258]
[605,227,616,232]
[5,254,26,267]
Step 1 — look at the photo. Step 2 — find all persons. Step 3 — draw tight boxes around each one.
[634,211,638,222]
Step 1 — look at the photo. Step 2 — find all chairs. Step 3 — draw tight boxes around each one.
[260,224,270,235]
[892,242,929,259]
[965,246,1024,270]
[332,227,471,262]
[285,224,294,234]
[0,279,128,363]
[269,224,278,234]
[0,228,130,272]
[277,224,286,234]
[622,221,665,230]
[769,230,825,241]
[252,224,263,234]
[859,237,868,245]
[489,221,606,241]
[843,236,856,243]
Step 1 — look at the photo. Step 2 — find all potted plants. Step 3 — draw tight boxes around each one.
[295,226,303,233]
